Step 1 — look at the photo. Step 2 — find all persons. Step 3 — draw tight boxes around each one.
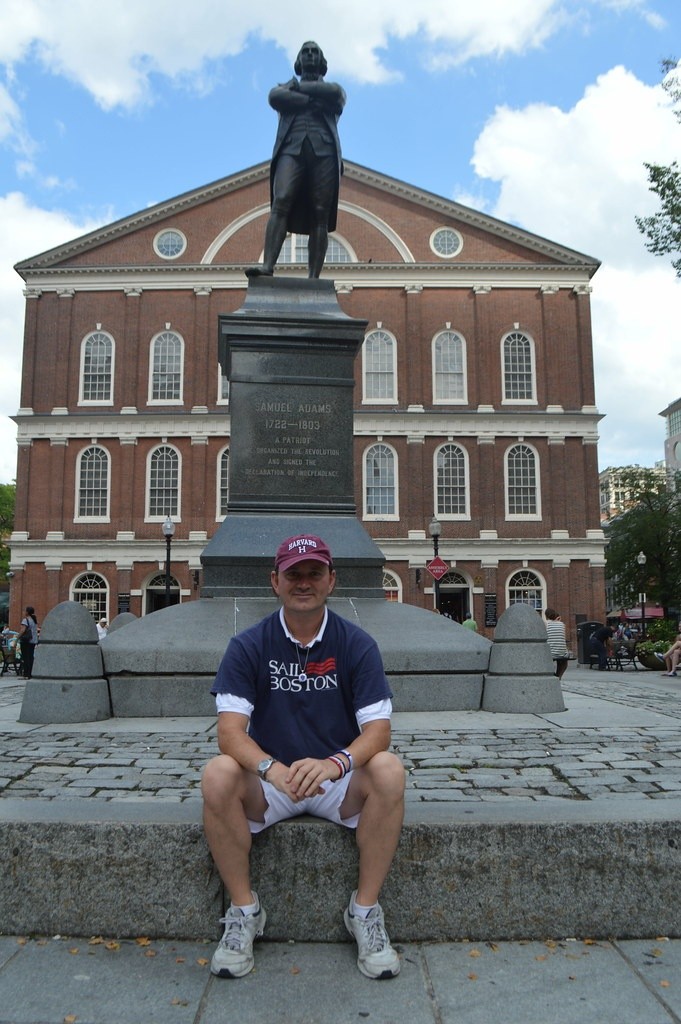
[590,621,641,670]
[544,608,568,658]
[462,612,478,632]
[2,624,9,633]
[244,41,346,279]
[13,607,38,679]
[662,622,681,676]
[654,641,681,663]
[201,535,405,980]
[96,617,108,640]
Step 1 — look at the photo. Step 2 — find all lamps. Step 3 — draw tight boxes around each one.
[6,572,15,577]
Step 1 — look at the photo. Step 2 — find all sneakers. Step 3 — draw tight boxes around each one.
[209,889,267,979]
[343,889,401,979]
[668,671,677,676]
[654,652,664,663]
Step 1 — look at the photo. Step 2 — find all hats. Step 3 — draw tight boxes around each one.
[99,618,108,623]
[273,532,334,573]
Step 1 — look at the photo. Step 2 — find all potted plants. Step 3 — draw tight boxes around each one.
[635,639,681,671]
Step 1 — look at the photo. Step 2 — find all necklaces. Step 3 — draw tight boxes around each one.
[286,620,323,682]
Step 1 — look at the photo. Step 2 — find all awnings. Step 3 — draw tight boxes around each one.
[606,607,663,617]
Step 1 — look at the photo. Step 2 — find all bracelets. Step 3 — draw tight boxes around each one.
[337,749,353,773]
[327,756,346,783]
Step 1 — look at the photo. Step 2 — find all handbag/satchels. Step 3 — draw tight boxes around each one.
[19,617,33,643]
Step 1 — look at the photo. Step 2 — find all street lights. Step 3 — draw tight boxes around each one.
[637,551,647,640]
[427,512,445,613]
[161,513,176,607]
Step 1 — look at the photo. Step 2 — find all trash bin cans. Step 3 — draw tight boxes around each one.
[578,621,604,664]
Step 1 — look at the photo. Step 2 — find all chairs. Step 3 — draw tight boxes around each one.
[0,643,20,677]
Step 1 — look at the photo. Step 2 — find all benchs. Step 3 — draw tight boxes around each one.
[590,641,638,671]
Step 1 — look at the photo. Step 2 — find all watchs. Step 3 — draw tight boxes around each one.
[257,758,279,783]
